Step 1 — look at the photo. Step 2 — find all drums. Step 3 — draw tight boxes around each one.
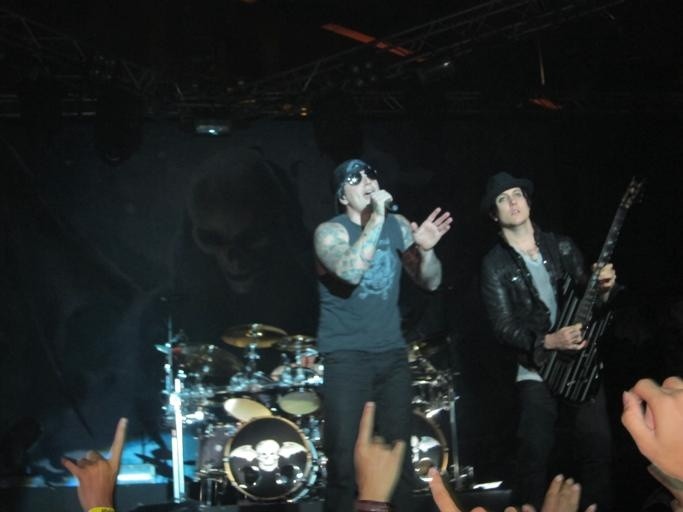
[275,363,320,417]
[224,416,319,503]
[223,369,277,422]
[407,408,448,493]
[198,422,242,479]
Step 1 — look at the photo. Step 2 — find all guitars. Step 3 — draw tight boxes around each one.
[532,176,644,407]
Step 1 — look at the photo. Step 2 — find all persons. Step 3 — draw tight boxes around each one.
[478,172,617,512]
[313,157,453,512]
[427,401,597,512]
[354,402,405,510]
[60,417,128,511]
[622,377,683,511]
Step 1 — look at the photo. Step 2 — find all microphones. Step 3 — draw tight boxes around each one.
[382,198,397,212]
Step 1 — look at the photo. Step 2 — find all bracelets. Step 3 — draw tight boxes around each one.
[353,500,392,511]
[87,506,114,511]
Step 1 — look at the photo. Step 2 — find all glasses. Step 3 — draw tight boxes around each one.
[348,168,379,184]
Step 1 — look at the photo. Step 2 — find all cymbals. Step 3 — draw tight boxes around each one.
[408,331,457,362]
[271,336,317,352]
[173,344,244,378]
[220,322,288,350]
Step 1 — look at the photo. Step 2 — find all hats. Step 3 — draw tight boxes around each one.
[332,158,369,214]
[481,171,534,213]
